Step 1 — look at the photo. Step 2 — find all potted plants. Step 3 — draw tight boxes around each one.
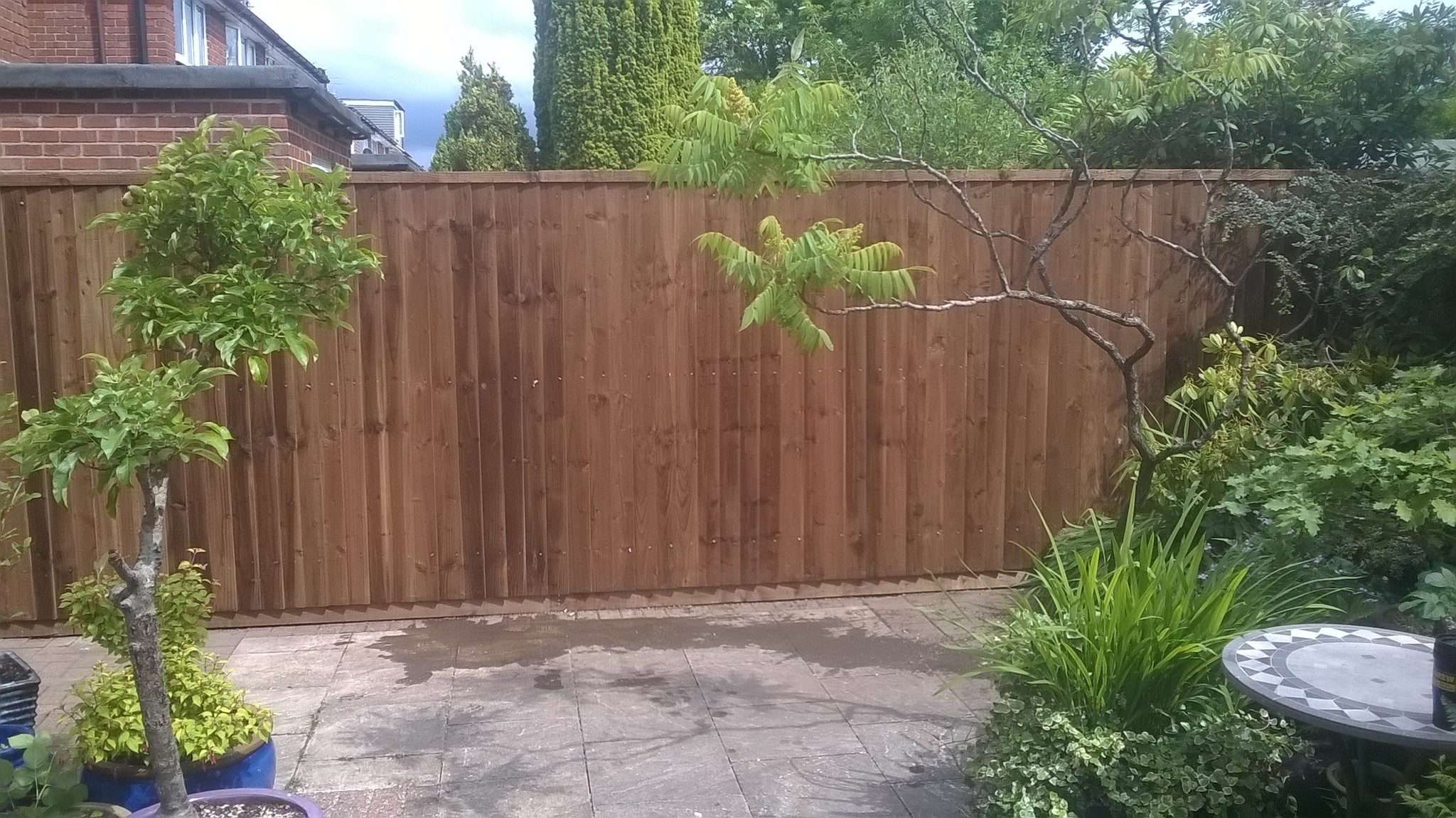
[0,113,324,818]
[56,547,281,814]
[0,359,45,732]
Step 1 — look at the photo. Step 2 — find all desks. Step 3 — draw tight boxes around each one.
[1220,622,1456,818]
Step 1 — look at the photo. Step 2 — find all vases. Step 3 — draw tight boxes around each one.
[1326,759,1406,814]
[0,724,35,765]
[0,731,132,818]
